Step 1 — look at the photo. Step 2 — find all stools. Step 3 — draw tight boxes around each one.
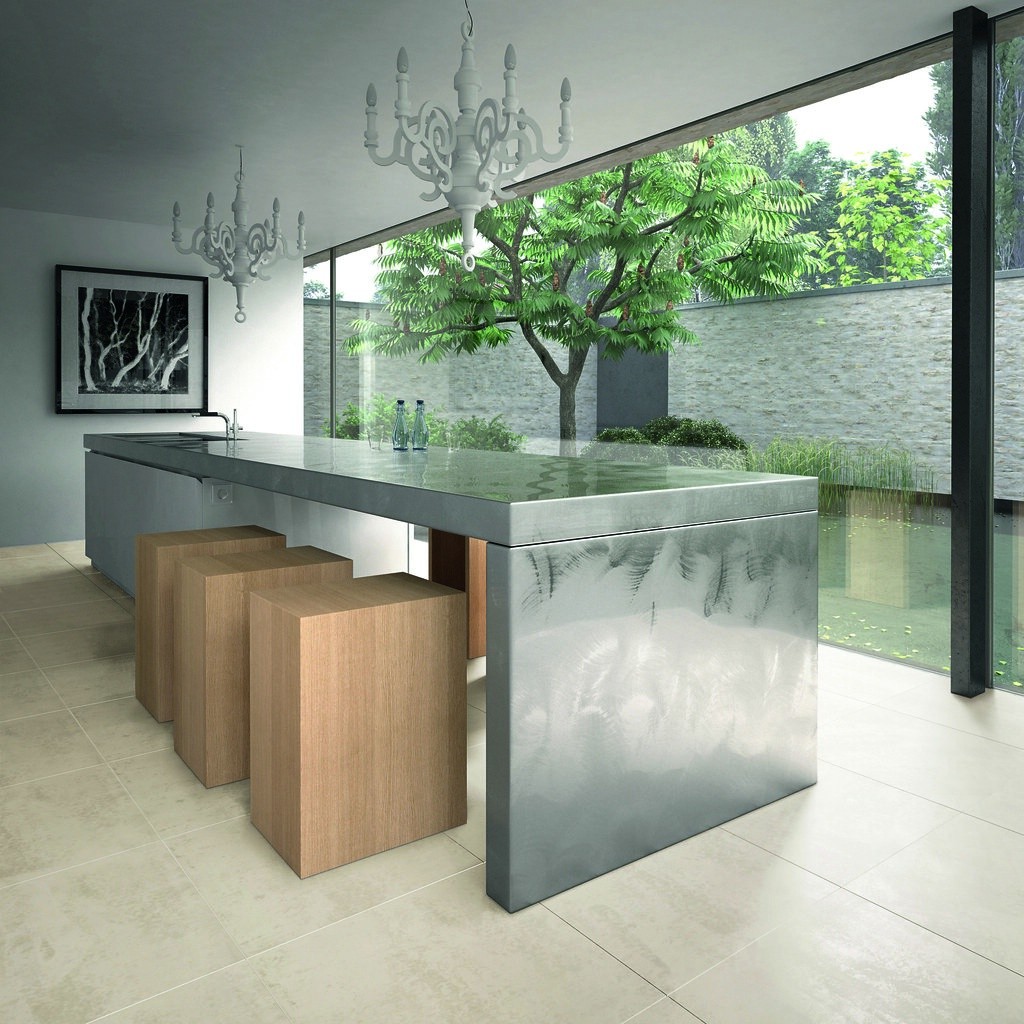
[133,523,287,725]
[250,571,467,879]
[174,546,353,789]
[425,527,487,660]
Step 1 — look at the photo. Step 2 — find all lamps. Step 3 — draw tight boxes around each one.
[170,145,305,323]
[363,0,574,272]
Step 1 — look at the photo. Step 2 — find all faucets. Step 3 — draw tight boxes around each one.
[190,411,230,441]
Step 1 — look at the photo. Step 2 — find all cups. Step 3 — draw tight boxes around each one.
[367,425,384,449]
[445,432,461,452]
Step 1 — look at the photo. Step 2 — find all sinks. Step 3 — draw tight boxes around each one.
[151,435,250,450]
[105,431,205,443]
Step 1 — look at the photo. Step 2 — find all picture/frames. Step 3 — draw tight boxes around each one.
[54,263,209,415]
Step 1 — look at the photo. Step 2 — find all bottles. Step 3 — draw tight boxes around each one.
[392,400,410,450]
[412,400,428,450]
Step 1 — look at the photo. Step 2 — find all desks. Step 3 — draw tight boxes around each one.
[82,431,819,913]
